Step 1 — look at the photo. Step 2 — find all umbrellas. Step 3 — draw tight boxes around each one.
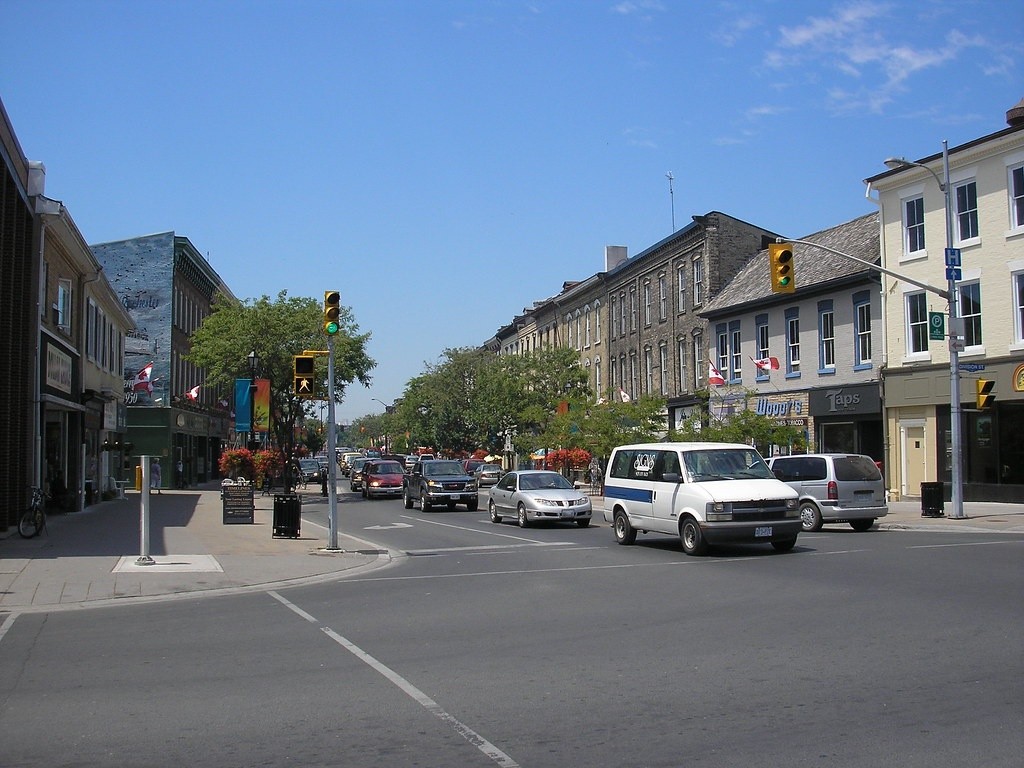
[536,447,553,470]
[531,454,545,464]
[485,454,502,464]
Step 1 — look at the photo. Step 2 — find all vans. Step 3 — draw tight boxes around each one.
[603,442,803,554]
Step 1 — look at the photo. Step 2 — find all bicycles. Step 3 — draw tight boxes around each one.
[295,472,307,490]
[17,486,52,538]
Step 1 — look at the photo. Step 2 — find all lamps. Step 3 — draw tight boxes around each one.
[114,440,119,445]
[104,439,108,444]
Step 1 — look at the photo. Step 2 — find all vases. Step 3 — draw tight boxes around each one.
[232,459,249,472]
[267,460,280,470]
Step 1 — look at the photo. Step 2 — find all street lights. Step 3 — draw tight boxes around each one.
[247,346,260,485]
[883,138,968,520]
[371,398,388,454]
[977,379,997,410]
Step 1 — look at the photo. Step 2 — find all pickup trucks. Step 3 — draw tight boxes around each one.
[403,459,479,512]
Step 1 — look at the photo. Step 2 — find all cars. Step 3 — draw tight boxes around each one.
[360,460,408,500]
[473,464,503,488]
[453,458,487,476]
[299,459,322,484]
[313,451,329,475]
[487,469,593,527]
[335,447,435,492]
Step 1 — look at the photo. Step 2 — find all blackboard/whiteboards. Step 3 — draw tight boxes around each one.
[223,485,254,524]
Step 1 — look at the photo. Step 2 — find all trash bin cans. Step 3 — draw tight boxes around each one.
[584,473,591,484]
[272,493,302,539]
[920,482,944,518]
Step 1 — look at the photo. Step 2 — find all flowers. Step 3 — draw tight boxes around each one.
[218,447,255,479]
[256,447,286,477]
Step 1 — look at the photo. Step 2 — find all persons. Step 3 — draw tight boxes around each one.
[533,459,543,470]
[175,460,183,487]
[149,457,162,494]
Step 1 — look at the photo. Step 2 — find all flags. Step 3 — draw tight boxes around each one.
[134,363,153,390]
[619,387,632,403]
[750,357,780,370]
[231,410,236,422]
[145,377,160,397]
[185,384,199,401]
[707,361,725,386]
[221,398,229,407]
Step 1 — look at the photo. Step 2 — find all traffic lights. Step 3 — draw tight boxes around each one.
[293,354,314,396]
[768,243,796,295]
[323,291,339,335]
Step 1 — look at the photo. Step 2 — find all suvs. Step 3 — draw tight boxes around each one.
[748,453,889,532]
